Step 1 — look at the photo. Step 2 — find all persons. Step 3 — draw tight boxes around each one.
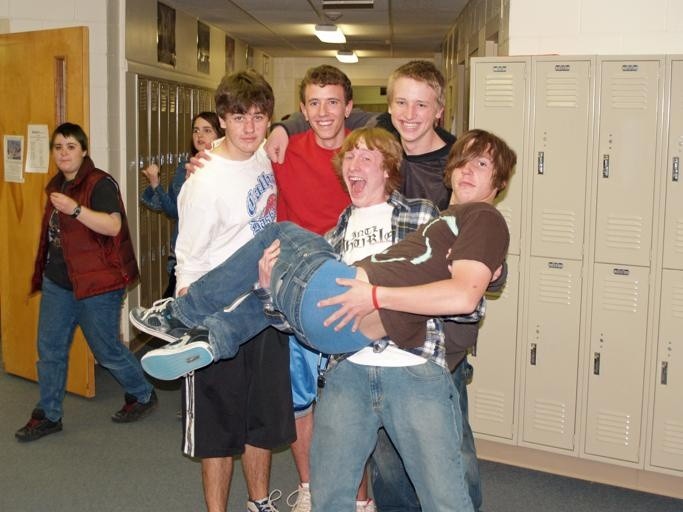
[12,122,159,442]
[172,67,297,511]
[183,62,382,511]
[258,126,508,512]
[128,127,516,382]
[263,61,483,512]
[140,109,225,297]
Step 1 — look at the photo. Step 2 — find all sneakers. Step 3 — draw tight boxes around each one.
[111,390,159,424]
[15,408,63,442]
[356,498,377,512]
[129,297,191,343]
[246,488,283,512]
[140,329,215,382]
[285,487,312,512]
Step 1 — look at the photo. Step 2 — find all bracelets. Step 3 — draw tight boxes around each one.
[372,285,381,310]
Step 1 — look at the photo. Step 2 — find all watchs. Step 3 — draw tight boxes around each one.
[69,202,82,220]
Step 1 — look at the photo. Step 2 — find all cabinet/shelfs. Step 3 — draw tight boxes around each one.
[135,70,222,350]
[455,53,683,501]
